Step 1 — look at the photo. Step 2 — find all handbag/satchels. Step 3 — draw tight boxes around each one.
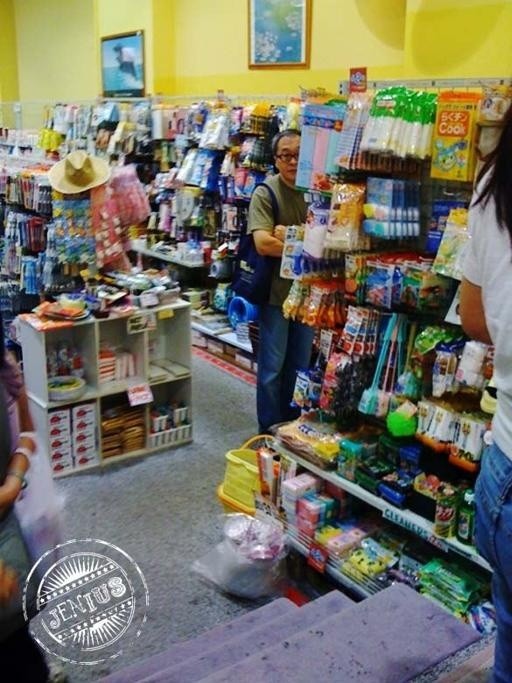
[232,182,281,310]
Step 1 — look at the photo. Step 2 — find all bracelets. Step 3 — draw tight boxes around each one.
[18,432,38,452]
[14,448,32,465]
[6,472,28,490]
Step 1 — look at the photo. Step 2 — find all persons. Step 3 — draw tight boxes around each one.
[246,129,315,434]
[0,343,39,631]
[455,99,512,683]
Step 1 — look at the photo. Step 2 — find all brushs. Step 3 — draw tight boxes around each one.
[357,314,407,419]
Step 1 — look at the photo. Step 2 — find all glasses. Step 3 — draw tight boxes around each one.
[276,152,299,164]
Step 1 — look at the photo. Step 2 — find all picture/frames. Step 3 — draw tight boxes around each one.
[247,0,312,70]
[99,28,146,99]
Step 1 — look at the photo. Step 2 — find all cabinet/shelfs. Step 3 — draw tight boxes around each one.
[267,412,494,640]
[18,293,195,478]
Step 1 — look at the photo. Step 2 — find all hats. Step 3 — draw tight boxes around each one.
[48,150,112,195]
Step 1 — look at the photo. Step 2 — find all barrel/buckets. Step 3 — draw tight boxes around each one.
[227,295,260,330]
